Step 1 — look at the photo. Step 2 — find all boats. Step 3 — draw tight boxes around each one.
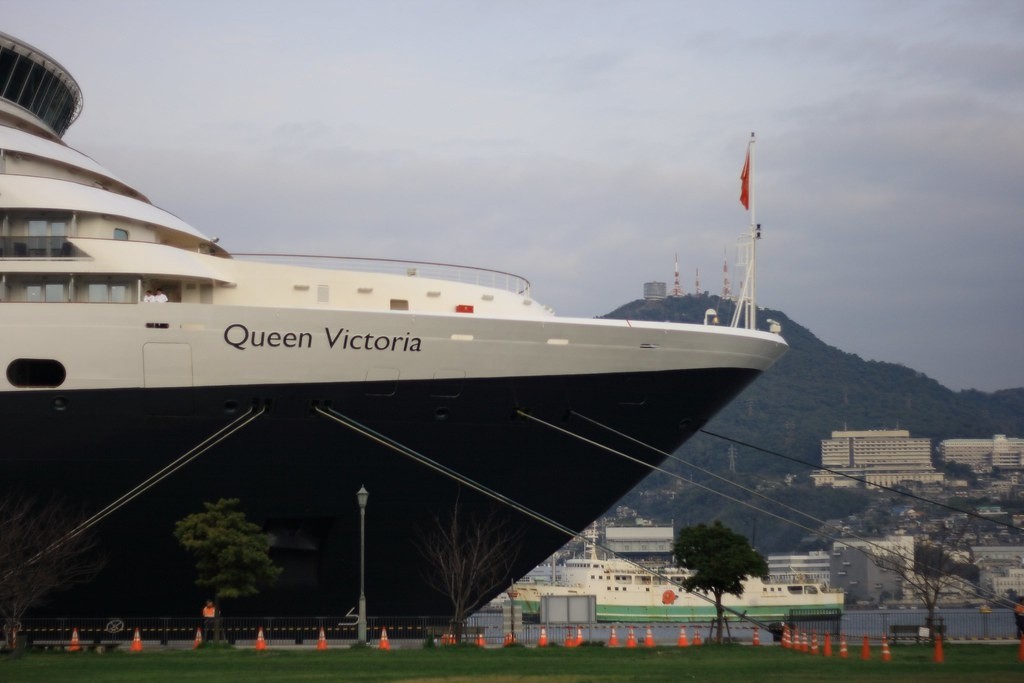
[879,604,887,610]
[2,29,791,640]
[910,605,917,609]
[483,525,845,622]
[899,605,906,609]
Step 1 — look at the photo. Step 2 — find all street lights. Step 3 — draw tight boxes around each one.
[356,483,370,648]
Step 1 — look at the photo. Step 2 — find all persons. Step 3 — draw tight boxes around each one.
[154,288,168,302]
[1013,595,1024,639]
[143,290,155,302]
[201,599,217,640]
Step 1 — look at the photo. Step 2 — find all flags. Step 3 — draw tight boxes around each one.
[740,149,749,210]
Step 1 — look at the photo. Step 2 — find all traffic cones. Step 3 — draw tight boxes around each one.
[781,625,819,655]
[643,626,656,647]
[440,633,456,644]
[537,625,547,647]
[881,634,891,661]
[839,632,849,658]
[255,627,266,649]
[626,625,637,647]
[692,626,702,645]
[1019,635,1024,660]
[316,627,327,650]
[677,625,688,647]
[69,628,80,651]
[130,628,143,652]
[608,626,619,647]
[935,635,943,662]
[565,626,584,648]
[378,626,390,650]
[753,626,760,646]
[192,628,202,650]
[824,632,832,656]
[861,635,871,660]
[502,633,515,646]
[476,634,484,646]
